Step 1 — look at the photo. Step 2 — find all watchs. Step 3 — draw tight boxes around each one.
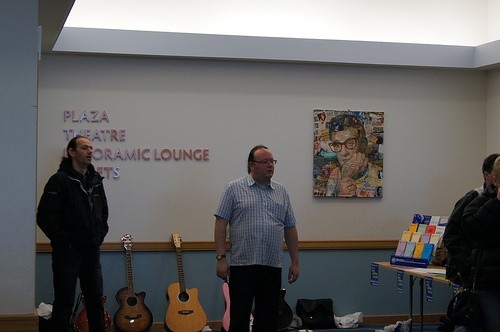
[216,254,226,260]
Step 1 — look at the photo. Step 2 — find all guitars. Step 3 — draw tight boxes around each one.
[220,280,254,332]
[113,233,154,332]
[275,288,294,330]
[74,293,112,332]
[164,231,208,332]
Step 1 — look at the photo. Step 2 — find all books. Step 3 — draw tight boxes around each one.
[395,212,449,262]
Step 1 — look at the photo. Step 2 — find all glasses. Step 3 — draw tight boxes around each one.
[251,160,277,165]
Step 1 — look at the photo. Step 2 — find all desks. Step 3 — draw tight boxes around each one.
[372,262,452,332]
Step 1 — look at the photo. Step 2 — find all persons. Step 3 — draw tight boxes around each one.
[462,156,500,332]
[442,153,500,332]
[35,136,110,332]
[212,145,300,332]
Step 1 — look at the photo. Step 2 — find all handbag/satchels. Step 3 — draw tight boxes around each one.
[437,290,487,332]
[296,298,337,329]
